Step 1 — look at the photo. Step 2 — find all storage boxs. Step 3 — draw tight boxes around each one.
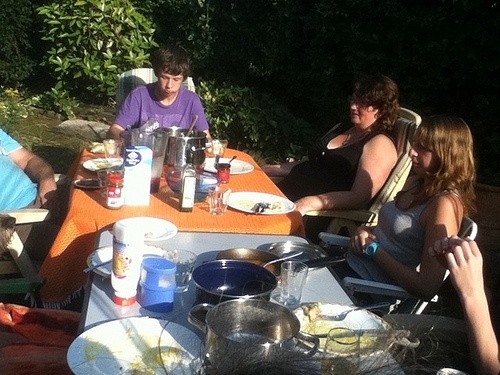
[123,146,154,206]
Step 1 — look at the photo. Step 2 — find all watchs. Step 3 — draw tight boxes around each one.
[365,241,380,258]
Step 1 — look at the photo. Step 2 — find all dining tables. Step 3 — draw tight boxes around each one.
[77,231,406,375]
[71,148,303,286]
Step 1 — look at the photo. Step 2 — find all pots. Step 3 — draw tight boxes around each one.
[156,126,207,167]
[186,298,319,368]
[192,250,304,321]
[216,247,348,278]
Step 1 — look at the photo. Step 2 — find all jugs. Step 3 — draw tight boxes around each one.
[131,128,168,194]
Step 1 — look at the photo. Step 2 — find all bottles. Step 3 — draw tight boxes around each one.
[107,221,146,306]
[177,150,197,213]
[216,163,231,184]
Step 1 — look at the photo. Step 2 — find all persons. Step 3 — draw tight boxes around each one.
[331,114,475,317]
[388,234,500,374]
[0,128,70,257]
[107,46,212,143]
[259,72,398,246]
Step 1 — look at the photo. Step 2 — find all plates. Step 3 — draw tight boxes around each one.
[66,316,204,375]
[256,241,331,272]
[293,302,397,358]
[87,244,168,279]
[204,157,254,175]
[74,157,123,190]
[119,217,178,242]
[229,191,297,215]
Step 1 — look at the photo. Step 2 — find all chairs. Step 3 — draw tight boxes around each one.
[117,68,196,110]
[319,217,478,314]
[319,107,423,235]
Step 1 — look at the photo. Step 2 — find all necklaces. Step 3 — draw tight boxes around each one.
[342,132,365,145]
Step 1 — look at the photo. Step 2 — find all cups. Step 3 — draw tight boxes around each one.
[321,326,361,375]
[138,257,177,313]
[206,186,232,216]
[279,260,308,309]
[101,131,165,210]
[212,140,228,157]
[162,249,197,294]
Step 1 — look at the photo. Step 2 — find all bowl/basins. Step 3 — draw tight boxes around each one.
[166,169,219,202]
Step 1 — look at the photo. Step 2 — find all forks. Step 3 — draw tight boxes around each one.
[322,302,392,322]
